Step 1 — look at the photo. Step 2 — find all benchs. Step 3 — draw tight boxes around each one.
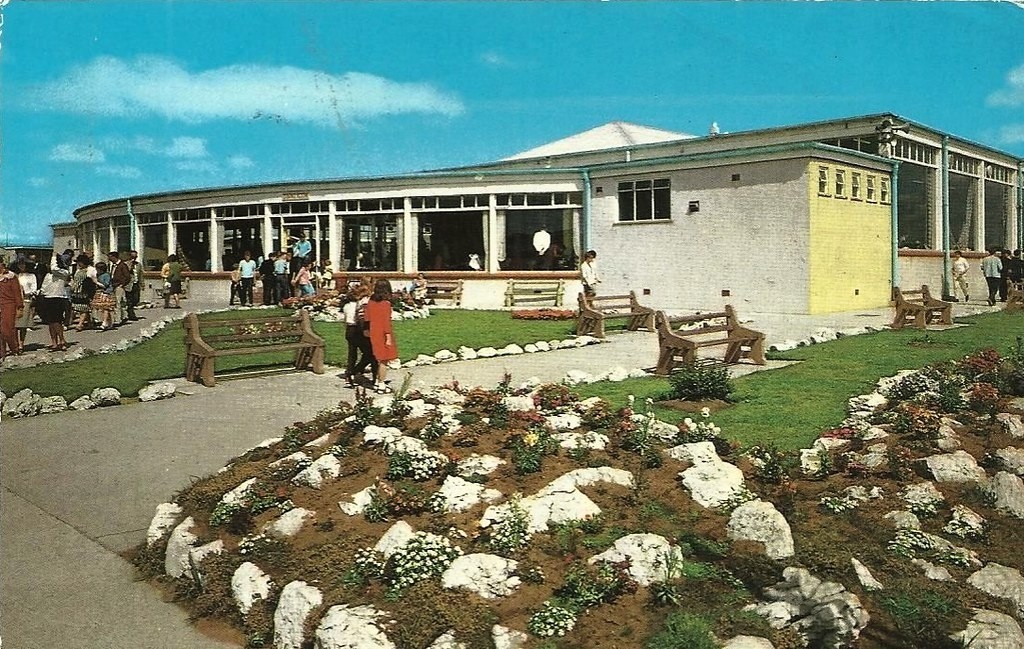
[576,289,656,338]
[654,303,767,375]
[410,279,463,306]
[892,283,953,328]
[503,277,565,307]
[1006,279,1023,309]
[181,306,327,386]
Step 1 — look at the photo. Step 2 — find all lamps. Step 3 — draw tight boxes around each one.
[688,200,699,211]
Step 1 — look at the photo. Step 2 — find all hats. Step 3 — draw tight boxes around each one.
[8,254,36,272]
[57,254,73,269]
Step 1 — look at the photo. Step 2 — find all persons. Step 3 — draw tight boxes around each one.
[581,251,601,307]
[0,246,182,363]
[952,251,969,302]
[532,223,566,273]
[230,232,428,395]
[981,247,1024,306]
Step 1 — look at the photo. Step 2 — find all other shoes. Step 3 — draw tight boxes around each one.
[19,346,25,354]
[5,350,13,355]
[129,317,139,322]
[62,322,114,331]
[345,370,355,386]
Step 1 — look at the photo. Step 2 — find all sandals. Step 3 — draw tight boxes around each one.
[61,344,67,351]
[374,386,396,393]
[49,346,59,351]
